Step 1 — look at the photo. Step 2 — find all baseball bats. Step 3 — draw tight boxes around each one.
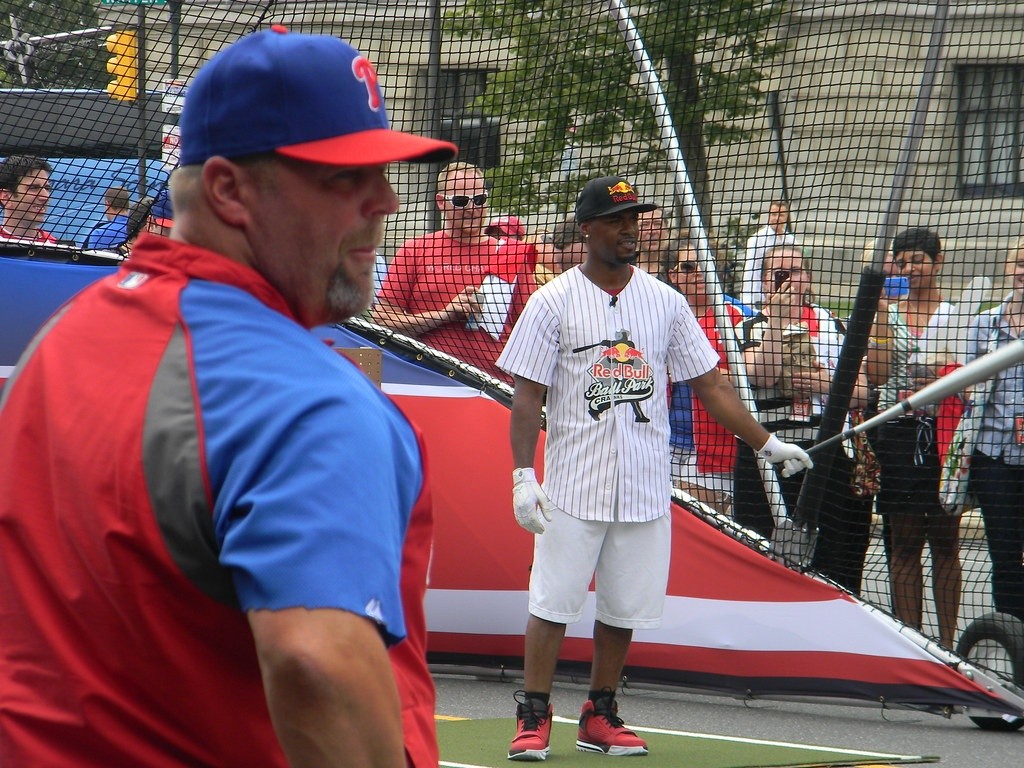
[773,340,1024,476]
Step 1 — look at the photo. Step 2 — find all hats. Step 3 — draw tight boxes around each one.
[180,27,458,168]
[576,175,658,225]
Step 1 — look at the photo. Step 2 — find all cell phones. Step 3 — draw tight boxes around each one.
[774,270,790,294]
[883,277,909,296]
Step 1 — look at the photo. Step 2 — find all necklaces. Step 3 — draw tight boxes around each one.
[1009,306,1018,334]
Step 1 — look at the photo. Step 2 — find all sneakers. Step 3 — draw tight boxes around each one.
[506,688,553,762]
[576,687,649,756]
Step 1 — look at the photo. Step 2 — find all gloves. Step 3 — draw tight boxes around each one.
[754,434,815,478]
[512,467,553,535]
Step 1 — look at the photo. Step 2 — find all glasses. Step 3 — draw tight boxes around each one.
[674,257,717,274]
[439,191,489,209]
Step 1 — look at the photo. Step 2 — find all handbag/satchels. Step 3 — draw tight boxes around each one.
[940,326,1000,514]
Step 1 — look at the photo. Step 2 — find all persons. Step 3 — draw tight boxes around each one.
[962,239,1024,620]
[740,201,797,308]
[552,217,588,272]
[87,185,130,250]
[0,22,458,768]
[485,214,556,288]
[493,176,814,759]
[372,162,535,384]
[660,229,758,478]
[632,204,682,293]
[116,197,154,258]
[1,154,56,243]
[733,244,874,597]
[862,228,962,652]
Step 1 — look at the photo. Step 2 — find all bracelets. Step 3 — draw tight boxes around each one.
[869,338,892,346]
